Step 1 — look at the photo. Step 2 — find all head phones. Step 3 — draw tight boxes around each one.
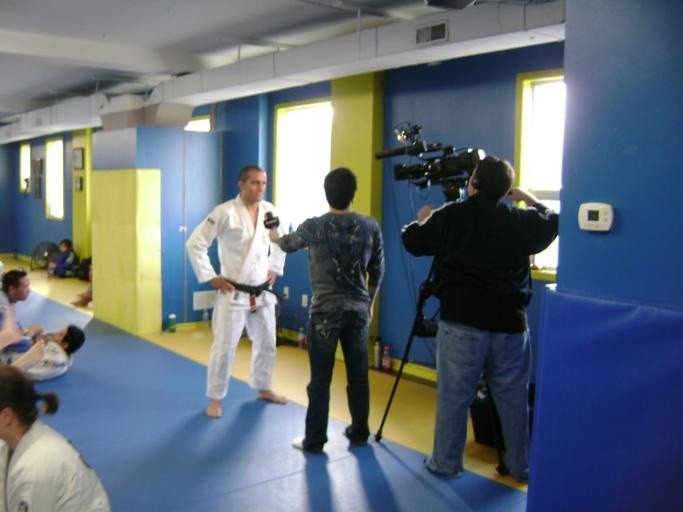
[471,161,482,189]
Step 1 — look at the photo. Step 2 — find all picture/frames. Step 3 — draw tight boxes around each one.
[72,147,84,170]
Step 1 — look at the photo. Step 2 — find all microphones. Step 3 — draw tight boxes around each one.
[264,212,279,228]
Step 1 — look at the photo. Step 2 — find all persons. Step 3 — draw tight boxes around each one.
[0,270,30,347]
[186,166,288,417]
[70,285,92,306]
[270,168,385,453]
[46,239,79,277]
[0,363,111,512]
[4,325,86,367]
[401,155,560,483]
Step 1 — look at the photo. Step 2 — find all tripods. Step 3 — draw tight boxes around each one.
[375,187,467,442]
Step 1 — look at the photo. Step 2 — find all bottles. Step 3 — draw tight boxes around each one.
[373,337,393,374]
[168,313,176,334]
[202,307,209,329]
[297,326,306,348]
